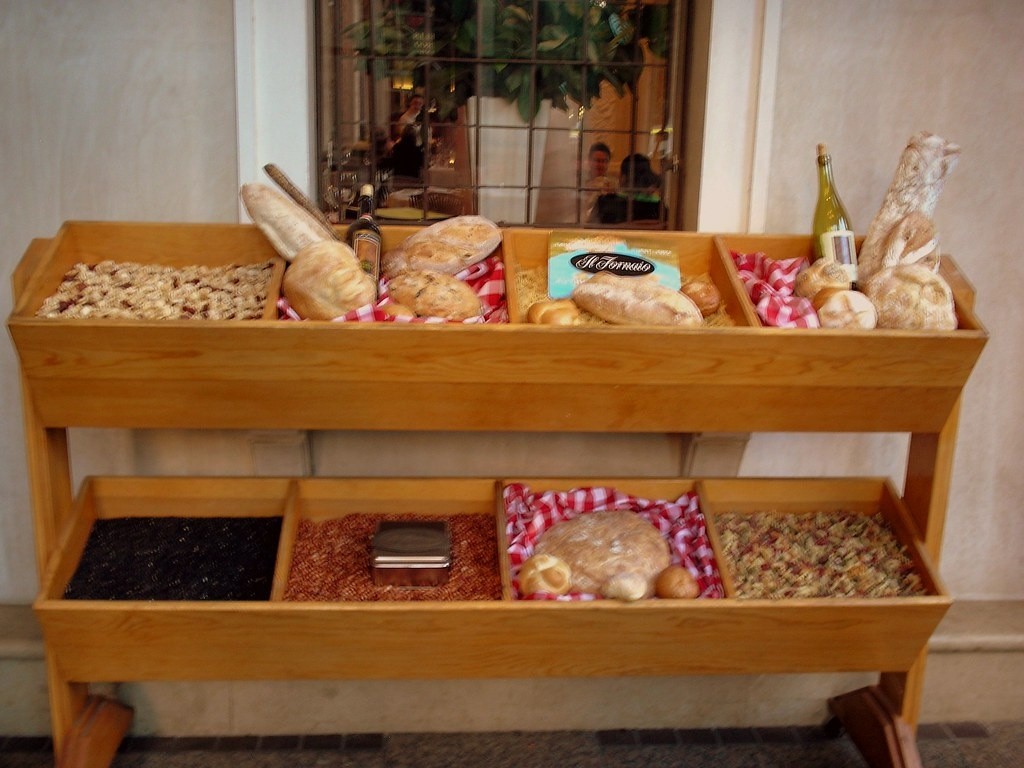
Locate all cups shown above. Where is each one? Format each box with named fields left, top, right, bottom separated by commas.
left=323, top=140, right=386, bottom=219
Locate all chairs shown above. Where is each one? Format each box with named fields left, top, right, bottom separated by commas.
left=410, top=194, right=464, bottom=215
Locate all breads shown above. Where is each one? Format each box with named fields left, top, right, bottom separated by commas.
left=797, top=129, right=962, bottom=329
left=516, top=509, right=700, bottom=599
left=527, top=278, right=722, bottom=329
left=238, top=181, right=499, bottom=322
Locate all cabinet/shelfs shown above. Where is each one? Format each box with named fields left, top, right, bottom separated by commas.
left=5, top=221, right=990, bottom=768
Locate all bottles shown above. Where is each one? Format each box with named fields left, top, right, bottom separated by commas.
left=811, top=142, right=858, bottom=287
left=346, top=183, right=383, bottom=290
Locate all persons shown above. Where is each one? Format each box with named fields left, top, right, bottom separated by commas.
left=583, top=152, right=670, bottom=231
left=395, top=94, right=425, bottom=146
left=646, top=130, right=669, bottom=160
left=580, top=140, right=621, bottom=212
left=352, top=121, right=425, bottom=180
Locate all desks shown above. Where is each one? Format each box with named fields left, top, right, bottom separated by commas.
left=387, top=186, right=456, bottom=209
left=428, top=165, right=454, bottom=187
